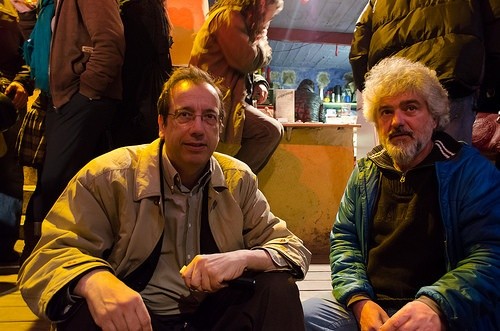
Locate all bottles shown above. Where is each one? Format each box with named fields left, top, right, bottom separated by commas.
left=327, top=90, right=351, bottom=102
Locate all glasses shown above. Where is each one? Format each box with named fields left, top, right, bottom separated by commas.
left=167, top=109, right=223, bottom=126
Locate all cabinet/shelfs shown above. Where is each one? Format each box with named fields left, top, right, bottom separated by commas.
left=322, top=93, right=357, bottom=119
left=258, top=120, right=365, bottom=265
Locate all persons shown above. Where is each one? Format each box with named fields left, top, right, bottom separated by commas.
left=0, top=0, right=172, bottom=269
left=18, top=64, right=312, bottom=331
left=182, top=0, right=285, bottom=177
left=302, top=56, right=500, bottom=331
left=348, top=0, right=500, bottom=146
left=294, top=79, right=326, bottom=123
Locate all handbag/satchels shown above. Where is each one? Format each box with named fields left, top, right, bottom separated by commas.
left=471, top=110, right=500, bottom=153
